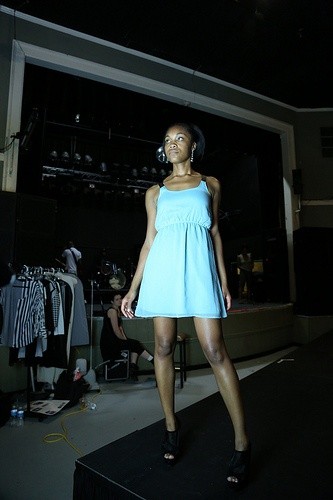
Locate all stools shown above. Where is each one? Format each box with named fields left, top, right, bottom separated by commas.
left=104, top=350, right=130, bottom=383
left=156, top=332, right=188, bottom=389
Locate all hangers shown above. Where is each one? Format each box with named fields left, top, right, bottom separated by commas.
left=12, top=265, right=65, bottom=288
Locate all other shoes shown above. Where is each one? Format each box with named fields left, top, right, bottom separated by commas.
left=130, top=368, right=137, bottom=381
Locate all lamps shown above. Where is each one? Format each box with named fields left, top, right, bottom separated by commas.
left=42, top=151, right=173, bottom=199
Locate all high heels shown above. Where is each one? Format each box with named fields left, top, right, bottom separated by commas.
left=224, top=441, right=252, bottom=488
left=161, top=429, right=181, bottom=466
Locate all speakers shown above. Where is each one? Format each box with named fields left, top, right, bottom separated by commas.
left=292, top=168, right=303, bottom=193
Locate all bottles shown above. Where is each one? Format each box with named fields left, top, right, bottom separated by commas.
left=17, top=407, right=24, bottom=426
left=11, top=405, right=18, bottom=425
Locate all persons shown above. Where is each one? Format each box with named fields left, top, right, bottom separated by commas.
left=231, top=248, right=255, bottom=306
left=120, top=123, right=256, bottom=488
left=100, top=292, right=154, bottom=382
left=61, top=240, right=82, bottom=277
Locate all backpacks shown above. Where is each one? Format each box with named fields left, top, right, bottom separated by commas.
left=53, top=369, right=89, bottom=409
